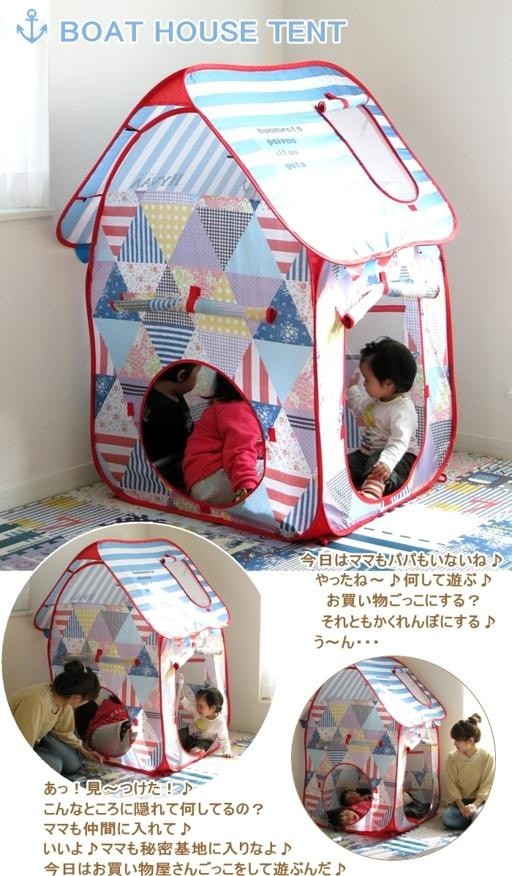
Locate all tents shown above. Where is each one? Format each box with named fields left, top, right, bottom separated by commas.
left=303, top=666, right=439, bottom=829
left=33, top=543, right=232, bottom=768
left=57, top=64, right=455, bottom=540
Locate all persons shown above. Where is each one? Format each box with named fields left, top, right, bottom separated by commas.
left=327, top=802, right=370, bottom=826
left=176, top=687, right=232, bottom=761
left=9, top=661, right=103, bottom=776
left=340, top=788, right=370, bottom=806
left=140, top=359, right=201, bottom=489
left=89, top=695, right=131, bottom=758
left=344, top=337, right=420, bottom=498
left=184, top=372, right=262, bottom=508
left=75, top=690, right=101, bottom=738
left=441, top=713, right=495, bottom=830
